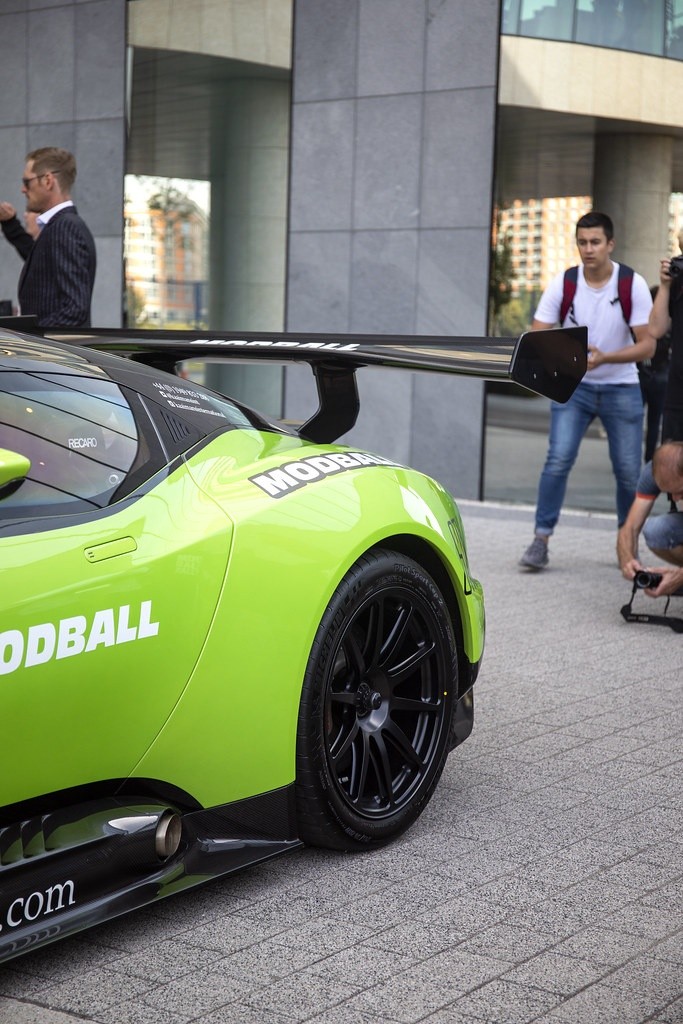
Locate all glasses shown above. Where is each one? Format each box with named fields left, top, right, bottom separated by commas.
left=22, top=170, right=60, bottom=189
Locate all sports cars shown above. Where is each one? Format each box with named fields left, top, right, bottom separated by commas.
left=0, top=324, right=591, bottom=962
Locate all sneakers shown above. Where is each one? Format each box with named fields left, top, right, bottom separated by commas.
left=521, top=537, right=549, bottom=566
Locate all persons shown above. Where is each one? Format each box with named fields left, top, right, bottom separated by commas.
left=0, top=146, right=96, bottom=332
left=518, top=211, right=683, bottom=598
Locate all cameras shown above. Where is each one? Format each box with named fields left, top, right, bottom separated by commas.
left=670, top=257, right=683, bottom=278
left=633, top=570, right=662, bottom=589
